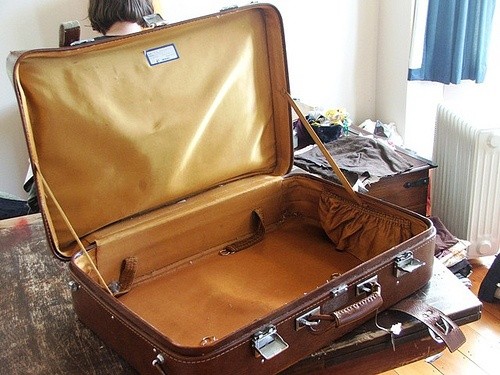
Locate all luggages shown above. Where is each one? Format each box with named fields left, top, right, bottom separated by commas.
left=11, top=1, right=500, bottom=365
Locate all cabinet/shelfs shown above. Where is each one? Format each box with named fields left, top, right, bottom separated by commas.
left=293, top=122, right=438, bottom=215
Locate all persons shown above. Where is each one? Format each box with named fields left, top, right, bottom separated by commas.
left=23, top=0, right=154, bottom=214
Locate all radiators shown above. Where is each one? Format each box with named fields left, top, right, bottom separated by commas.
left=430, top=103, right=500, bottom=258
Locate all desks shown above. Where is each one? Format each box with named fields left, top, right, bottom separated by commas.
left=1, top=165, right=483, bottom=375
